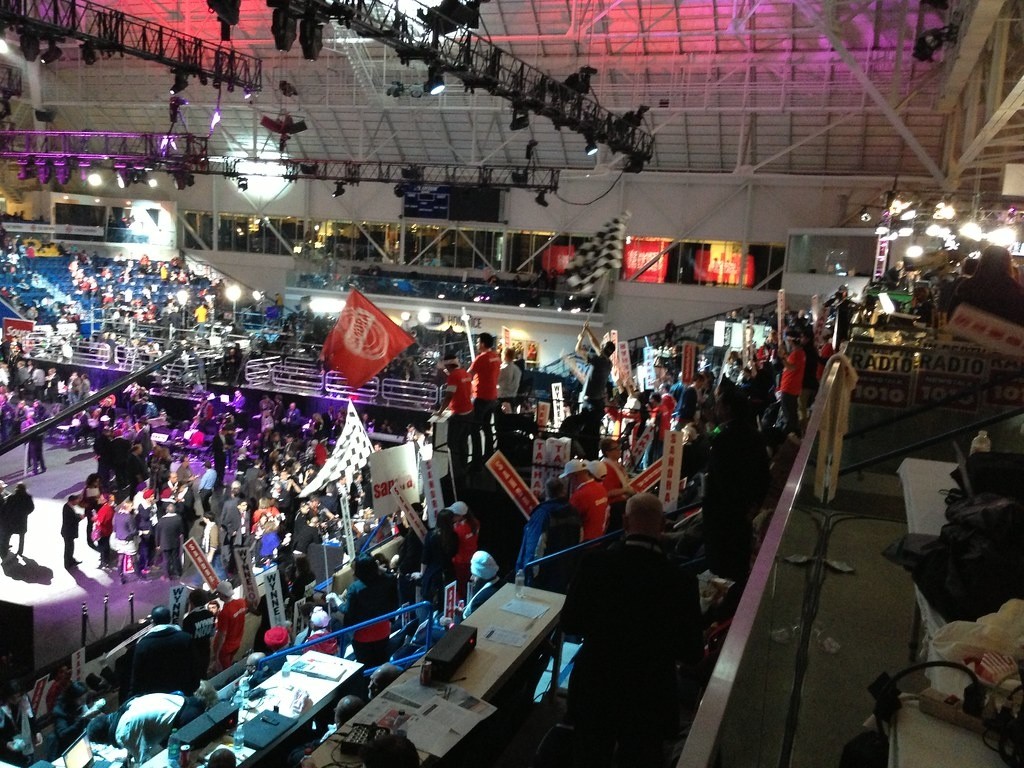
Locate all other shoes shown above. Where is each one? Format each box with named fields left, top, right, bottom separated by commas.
left=67, top=558, right=181, bottom=585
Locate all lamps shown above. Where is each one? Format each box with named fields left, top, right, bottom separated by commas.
left=0, top=1, right=646, bottom=208
left=913, top=22, right=956, bottom=65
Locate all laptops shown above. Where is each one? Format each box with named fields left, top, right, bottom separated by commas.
left=61, top=729, right=111, bottom=768
left=230, top=709, right=299, bottom=750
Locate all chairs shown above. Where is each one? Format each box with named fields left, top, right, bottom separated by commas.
left=0, top=255, right=208, bottom=326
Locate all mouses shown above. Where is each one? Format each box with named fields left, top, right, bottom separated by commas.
left=248, top=687, right=266, bottom=700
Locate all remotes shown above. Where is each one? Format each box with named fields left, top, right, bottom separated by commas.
left=261, top=715, right=280, bottom=726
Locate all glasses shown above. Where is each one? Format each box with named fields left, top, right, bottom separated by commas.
left=609, top=446, right=619, bottom=451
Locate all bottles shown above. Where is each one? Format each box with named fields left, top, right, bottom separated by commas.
left=458, top=599, right=464, bottom=611
left=234, top=691, right=243, bottom=719
left=300, top=749, right=315, bottom=768
left=234, top=728, right=243, bottom=755
left=515, top=570, right=525, bottom=598
left=241, top=680, right=249, bottom=704
left=168, top=728, right=179, bottom=768
left=969, top=431, right=991, bottom=457
left=325, top=532, right=329, bottom=543
left=282, top=662, right=289, bottom=685
left=393, top=710, right=408, bottom=737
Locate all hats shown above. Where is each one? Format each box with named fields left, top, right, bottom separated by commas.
left=265, top=627, right=291, bottom=649
left=217, top=581, right=233, bottom=597
left=583, top=460, right=607, bottom=480
left=310, top=605, right=329, bottom=628
left=0, top=480, right=8, bottom=489
left=143, top=489, right=154, bottom=498
left=359, top=734, right=419, bottom=768
left=470, top=551, right=499, bottom=579
left=161, top=488, right=172, bottom=498
left=247, top=652, right=265, bottom=665
left=151, top=606, right=172, bottom=625
left=445, top=501, right=468, bottom=515
left=558, top=459, right=588, bottom=479
left=100, top=415, right=110, bottom=422
left=136, top=482, right=148, bottom=490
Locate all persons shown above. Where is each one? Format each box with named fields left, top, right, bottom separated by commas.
left=1, top=210, right=1023, bottom=768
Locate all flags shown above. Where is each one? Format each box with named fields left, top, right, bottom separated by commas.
left=321, top=291, right=415, bottom=390
left=564, top=216, right=626, bottom=289
left=299, top=402, right=375, bottom=497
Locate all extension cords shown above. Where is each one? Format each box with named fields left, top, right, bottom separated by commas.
left=918, top=687, right=1024, bottom=746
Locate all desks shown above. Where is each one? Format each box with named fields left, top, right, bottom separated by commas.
left=305, top=542, right=344, bottom=584
left=137, top=649, right=365, bottom=768
left=897, top=457, right=975, bottom=700
left=300, top=583, right=568, bottom=768
